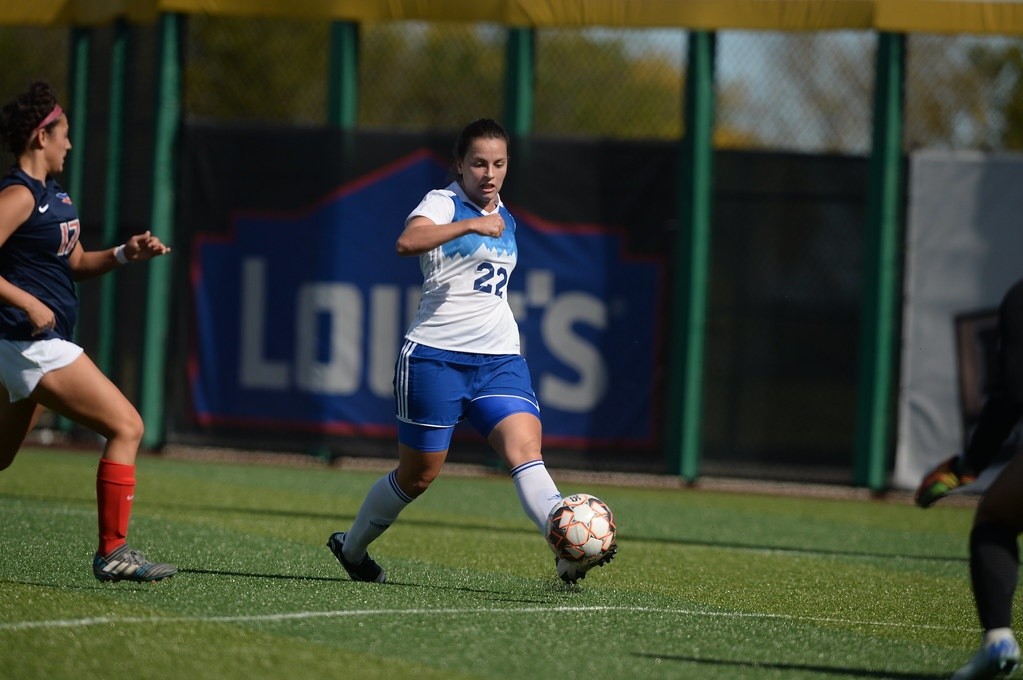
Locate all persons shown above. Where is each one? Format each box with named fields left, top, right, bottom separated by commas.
left=326, top=116, right=620, bottom=585
left=914, top=277, right=1023, bottom=680
left=0, top=82, right=184, bottom=582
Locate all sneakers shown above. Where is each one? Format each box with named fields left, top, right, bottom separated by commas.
left=328, top=532, right=384, bottom=583
left=555, top=543, right=619, bottom=583
left=93, top=544, right=178, bottom=582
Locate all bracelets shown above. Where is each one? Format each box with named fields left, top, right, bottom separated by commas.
left=113, top=244, right=129, bottom=264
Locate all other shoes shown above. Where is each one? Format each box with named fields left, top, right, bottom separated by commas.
left=951, top=626, right=1022, bottom=680
left=913, top=456, right=977, bottom=508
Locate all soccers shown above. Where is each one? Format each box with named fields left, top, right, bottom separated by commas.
left=542, top=492, right=618, bottom=568
